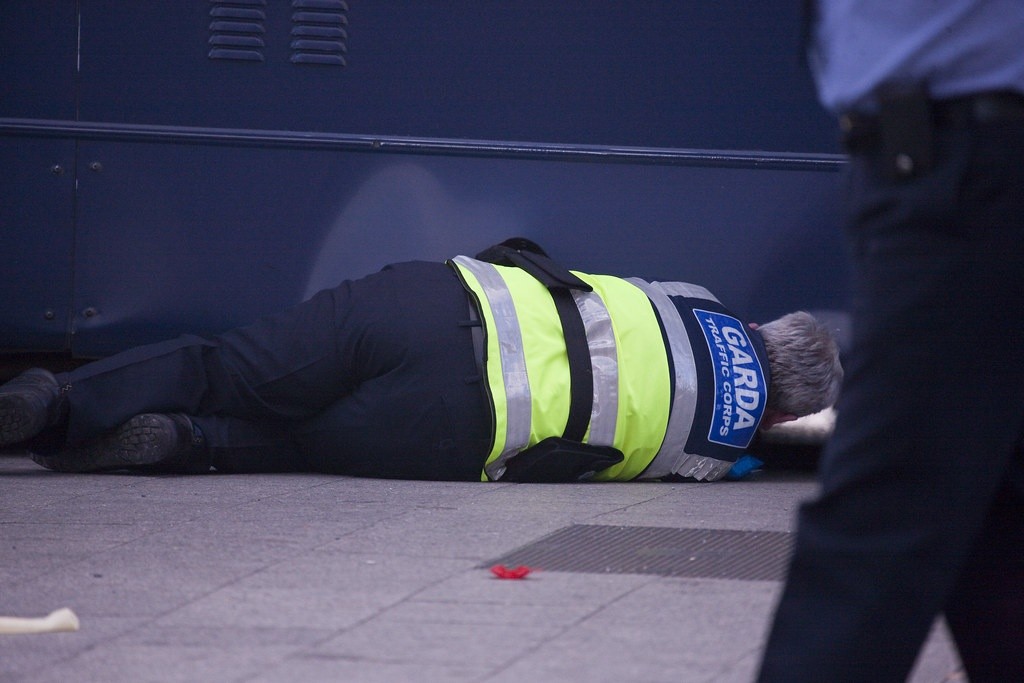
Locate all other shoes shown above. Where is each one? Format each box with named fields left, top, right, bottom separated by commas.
left=0, top=368, right=65, bottom=442
left=29, top=412, right=199, bottom=475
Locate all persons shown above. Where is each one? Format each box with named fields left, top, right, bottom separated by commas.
left=0, top=234, right=850, bottom=482
left=752, top=0, right=1024, bottom=683
